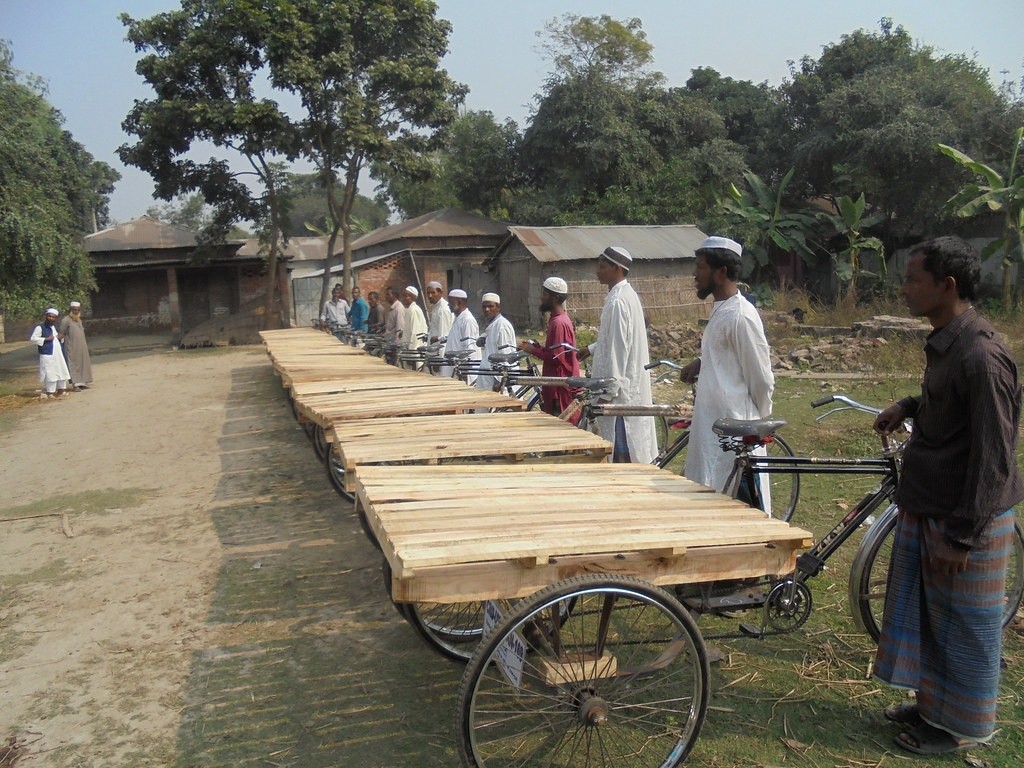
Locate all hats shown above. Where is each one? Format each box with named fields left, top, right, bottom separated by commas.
left=601, top=246, right=632, bottom=270
left=543, top=277, right=567, bottom=293
left=405, top=286, right=418, bottom=297
left=428, top=282, right=442, bottom=290
left=449, top=289, right=467, bottom=298
left=70, top=302, right=80, bottom=307
left=481, top=293, right=500, bottom=304
left=695, top=236, right=743, bottom=258
left=44, top=309, right=59, bottom=316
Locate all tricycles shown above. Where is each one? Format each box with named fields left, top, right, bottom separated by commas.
left=390, top=392, right=1023, bottom=768
left=271, top=316, right=808, bottom=539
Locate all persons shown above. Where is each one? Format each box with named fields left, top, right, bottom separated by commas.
left=30, top=308, right=71, bottom=399
left=320, top=283, right=523, bottom=415
left=872, top=236, right=1024, bottom=752
left=59, top=301, right=93, bottom=392
left=575, top=247, right=661, bottom=468
left=520, top=277, right=584, bottom=425
left=684, top=234, right=775, bottom=618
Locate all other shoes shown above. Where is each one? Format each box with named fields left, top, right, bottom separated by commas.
left=718, top=609, right=753, bottom=618
left=48, top=394, right=55, bottom=399
left=72, top=385, right=91, bottom=392
left=885, top=704, right=977, bottom=754
left=58, top=391, right=70, bottom=396
